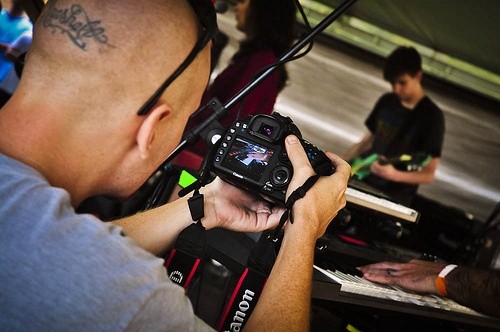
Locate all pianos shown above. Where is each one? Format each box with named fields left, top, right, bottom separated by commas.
left=172, top=227, right=500, bottom=330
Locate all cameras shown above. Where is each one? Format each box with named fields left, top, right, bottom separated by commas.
left=212, top=112, right=335, bottom=208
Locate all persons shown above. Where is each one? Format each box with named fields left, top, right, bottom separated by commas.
left=180, top=1, right=299, bottom=157
left=329, top=46, right=446, bottom=206
left=1, top=0, right=351, bottom=332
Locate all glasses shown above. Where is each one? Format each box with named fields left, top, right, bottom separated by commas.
left=137, top=0, right=217, bottom=116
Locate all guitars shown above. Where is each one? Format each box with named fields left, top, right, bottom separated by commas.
left=349, top=153, right=413, bottom=180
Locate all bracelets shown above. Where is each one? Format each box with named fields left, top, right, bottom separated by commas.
left=437, top=262, right=458, bottom=297
